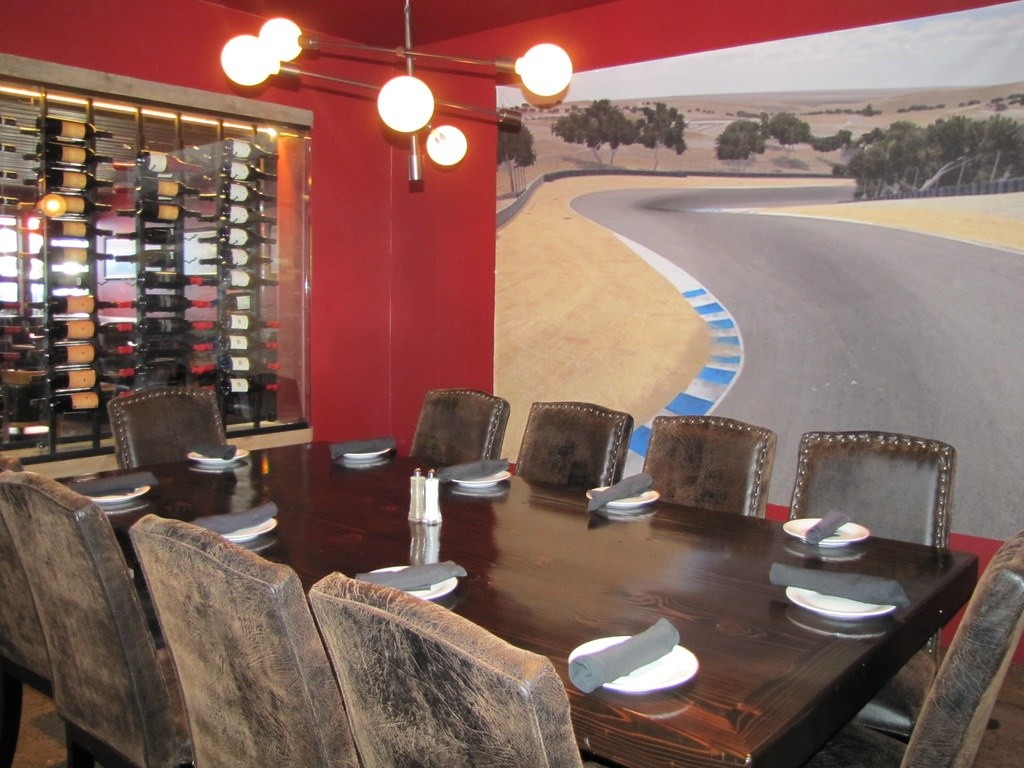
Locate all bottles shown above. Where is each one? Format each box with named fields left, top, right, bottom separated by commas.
left=25, top=273, right=115, bottom=414
left=113, top=150, right=202, bottom=266
left=198, top=206, right=279, bottom=289
left=18, top=116, right=114, bottom=261
left=0, top=117, right=22, bottom=359
left=408, top=468, right=426, bottom=523
left=200, top=138, right=279, bottom=204
left=197, top=356, right=280, bottom=398
left=116, top=270, right=201, bottom=397
left=422, top=468, right=443, bottom=524
left=197, top=293, right=279, bottom=354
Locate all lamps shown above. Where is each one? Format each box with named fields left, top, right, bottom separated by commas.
left=222, top=0, right=573, bottom=181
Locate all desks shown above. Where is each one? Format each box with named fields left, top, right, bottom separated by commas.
left=55, top=441, right=978, bottom=768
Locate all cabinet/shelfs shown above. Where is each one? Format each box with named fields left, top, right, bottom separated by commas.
left=0, top=52, right=315, bottom=466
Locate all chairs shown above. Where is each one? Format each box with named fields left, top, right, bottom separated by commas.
left=801, top=531, right=1024, bottom=768
left=406, top=388, right=510, bottom=463
left=107, top=386, right=227, bottom=469
left=642, top=415, right=777, bottom=518
left=0, top=454, right=582, bottom=768
left=789, top=431, right=957, bottom=739
left=515, top=401, right=635, bottom=490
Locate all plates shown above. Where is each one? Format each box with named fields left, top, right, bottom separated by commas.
left=372, top=566, right=458, bottom=599
left=783, top=518, right=870, bottom=546
left=221, top=518, right=278, bottom=541
left=188, top=449, right=249, bottom=465
left=786, top=585, right=896, bottom=618
left=586, top=486, right=660, bottom=508
left=568, top=635, right=699, bottom=692
left=89, top=485, right=151, bottom=503
left=452, top=470, right=511, bottom=487
left=343, top=448, right=391, bottom=460
